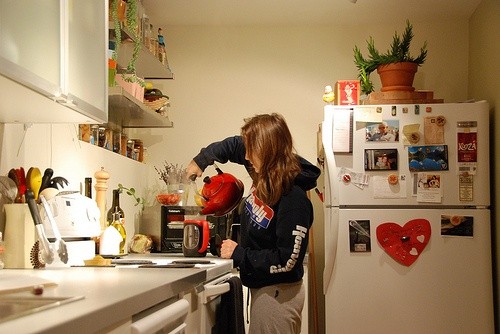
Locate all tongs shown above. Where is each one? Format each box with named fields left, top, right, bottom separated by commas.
left=26, top=187, right=69, bottom=265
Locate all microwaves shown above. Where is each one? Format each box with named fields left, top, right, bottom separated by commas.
left=160, top=206, right=234, bottom=254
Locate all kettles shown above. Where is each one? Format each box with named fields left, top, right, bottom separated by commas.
left=190, top=163, right=244, bottom=218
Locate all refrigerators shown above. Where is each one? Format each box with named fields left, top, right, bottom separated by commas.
left=313, top=104, right=497, bottom=334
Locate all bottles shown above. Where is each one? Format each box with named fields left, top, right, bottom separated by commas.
left=84, top=177, right=92, bottom=199
left=80, top=122, right=143, bottom=163
left=107, top=190, right=125, bottom=226
left=108, top=206, right=126, bottom=252
left=149, top=24, right=159, bottom=59
left=177, top=184, right=185, bottom=206
left=158, top=27, right=167, bottom=66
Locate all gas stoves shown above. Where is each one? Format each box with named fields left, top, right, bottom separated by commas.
left=41, top=194, right=234, bottom=284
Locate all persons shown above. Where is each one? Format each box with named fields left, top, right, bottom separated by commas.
left=375, top=156, right=392, bottom=169
left=186, top=113, right=321, bottom=334
left=367, top=122, right=395, bottom=141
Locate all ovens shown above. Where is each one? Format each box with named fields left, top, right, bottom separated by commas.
left=202, top=272, right=240, bottom=334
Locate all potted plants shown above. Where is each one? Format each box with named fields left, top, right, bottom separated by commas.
left=353, top=18, right=429, bottom=96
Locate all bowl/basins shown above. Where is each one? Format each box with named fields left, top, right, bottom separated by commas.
left=156, top=193, right=182, bottom=206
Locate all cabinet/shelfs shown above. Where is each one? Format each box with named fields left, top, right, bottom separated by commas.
left=0, top=0, right=110, bottom=125
left=108, top=20, right=174, bottom=128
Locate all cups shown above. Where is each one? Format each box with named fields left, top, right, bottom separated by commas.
left=183, top=214, right=209, bottom=257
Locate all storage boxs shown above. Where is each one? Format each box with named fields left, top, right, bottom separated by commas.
left=114, top=73, right=144, bottom=103
left=334, top=80, right=360, bottom=106
